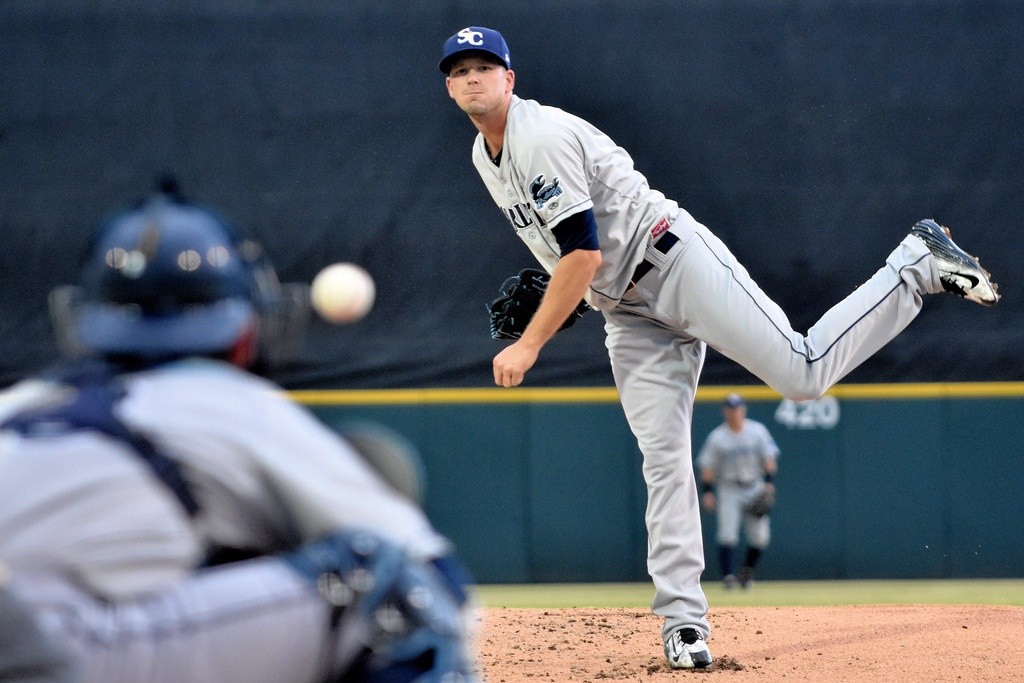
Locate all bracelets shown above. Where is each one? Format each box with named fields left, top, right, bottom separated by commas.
left=764, top=472, right=776, bottom=486
left=698, top=482, right=715, bottom=495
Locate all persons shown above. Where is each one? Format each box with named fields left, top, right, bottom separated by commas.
left=1, top=199, right=474, bottom=683
left=694, top=392, right=782, bottom=592
left=439, top=25, right=1003, bottom=670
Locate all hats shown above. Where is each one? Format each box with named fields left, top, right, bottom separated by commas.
left=439, top=26, right=510, bottom=76
left=73, top=200, right=250, bottom=355
left=723, top=395, right=744, bottom=408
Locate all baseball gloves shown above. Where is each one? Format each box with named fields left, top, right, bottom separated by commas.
left=483, top=268, right=593, bottom=342
left=747, top=492, right=776, bottom=519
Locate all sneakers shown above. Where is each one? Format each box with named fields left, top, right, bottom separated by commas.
left=910, top=219, right=1001, bottom=306
left=664, top=628, right=712, bottom=670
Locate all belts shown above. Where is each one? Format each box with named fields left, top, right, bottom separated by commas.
left=623, top=231, right=680, bottom=295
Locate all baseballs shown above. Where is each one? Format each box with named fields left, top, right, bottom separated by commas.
left=308, top=261, right=377, bottom=328
